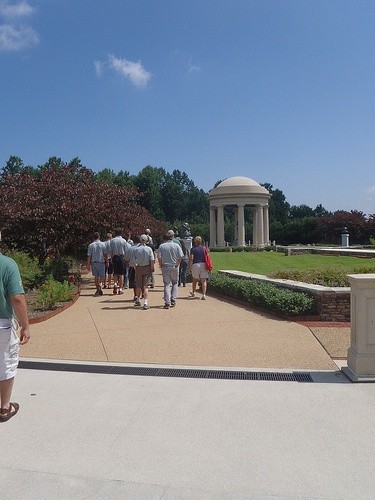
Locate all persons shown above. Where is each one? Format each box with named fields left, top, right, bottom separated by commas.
left=180, top=223, right=191, bottom=239
left=132, top=234, right=154, bottom=309
left=188, top=236, right=208, bottom=300
left=157, top=230, right=184, bottom=309
left=87, top=232, right=109, bottom=295
left=174, top=227, right=179, bottom=237
left=103, top=229, right=190, bottom=289
left=110, top=227, right=129, bottom=294
left=0, top=254, right=31, bottom=422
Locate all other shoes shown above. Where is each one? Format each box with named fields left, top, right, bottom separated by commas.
left=171, top=302, right=176, bottom=305
left=98, top=285, right=103, bottom=295
left=164, top=305, right=170, bottom=309
left=143, top=304, right=148, bottom=310
left=202, top=296, right=206, bottom=301
left=95, top=291, right=99, bottom=294
left=113, top=285, right=117, bottom=295
left=118, top=290, right=124, bottom=295
left=134, top=301, right=141, bottom=306
left=190, top=291, right=195, bottom=297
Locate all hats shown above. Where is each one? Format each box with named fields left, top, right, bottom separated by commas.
left=139, top=234, right=148, bottom=242
left=166, top=230, right=175, bottom=237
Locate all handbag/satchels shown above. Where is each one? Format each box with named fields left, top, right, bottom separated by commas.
left=203, top=247, right=213, bottom=272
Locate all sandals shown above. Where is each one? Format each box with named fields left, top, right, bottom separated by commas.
left=0, top=403, right=19, bottom=421
